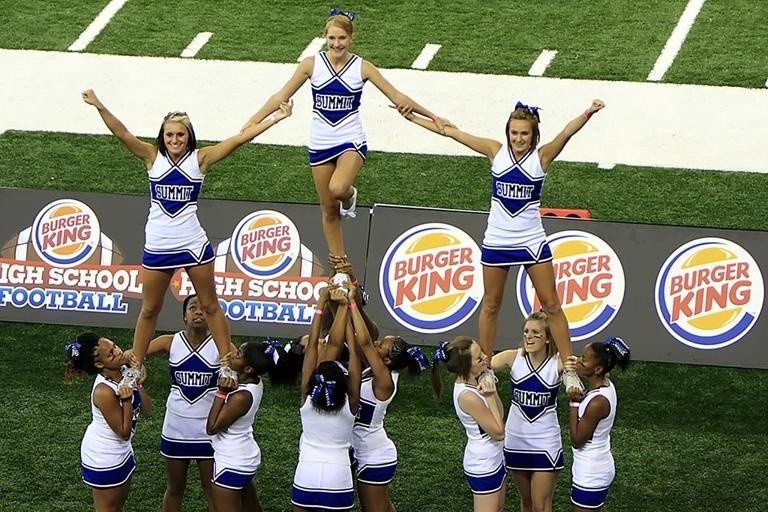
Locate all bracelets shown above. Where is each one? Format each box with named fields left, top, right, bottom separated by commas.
left=584, top=110, right=593, bottom=119
left=271, top=115, right=279, bottom=125
left=569, top=398, right=580, bottom=407
left=136, top=383, right=143, bottom=389
left=431, top=113, right=438, bottom=121
left=214, top=389, right=227, bottom=401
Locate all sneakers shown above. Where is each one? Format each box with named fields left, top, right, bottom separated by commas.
left=562, top=370, right=584, bottom=394
left=477, top=367, right=497, bottom=393
left=116, top=363, right=148, bottom=396
left=217, top=363, right=238, bottom=388
left=339, top=185, right=357, bottom=223
left=330, top=272, right=350, bottom=301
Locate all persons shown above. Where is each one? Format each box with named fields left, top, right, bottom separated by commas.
left=565, top=336, right=631, bottom=512
left=240, top=6, right=458, bottom=297
left=206, top=336, right=282, bottom=512
left=83, top=89, right=294, bottom=396
left=389, top=98, right=606, bottom=394
left=64, top=333, right=152, bottom=512
left=431, top=335, right=508, bottom=512
left=482, top=307, right=579, bottom=512
left=283, top=281, right=430, bottom=512
left=123, top=294, right=238, bottom=512
